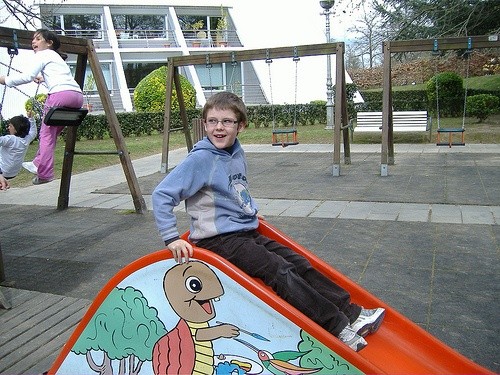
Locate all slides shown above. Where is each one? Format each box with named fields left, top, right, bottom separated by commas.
left=195, top=218, right=500, bottom=375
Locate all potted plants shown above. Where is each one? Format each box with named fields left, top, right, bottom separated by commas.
left=214, top=4, right=230, bottom=47
left=184, top=19, right=205, bottom=47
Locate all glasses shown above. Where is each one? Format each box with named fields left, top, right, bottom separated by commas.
left=206, top=119, right=241, bottom=125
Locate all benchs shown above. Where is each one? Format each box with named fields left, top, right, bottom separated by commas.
left=349, top=110, right=433, bottom=143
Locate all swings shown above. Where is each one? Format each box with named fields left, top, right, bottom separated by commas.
left=0, top=62, right=88, bottom=127
left=265, top=56, right=301, bottom=148
left=0, top=47, right=43, bottom=137
left=430, top=49, right=472, bottom=148
left=206, top=60, right=238, bottom=97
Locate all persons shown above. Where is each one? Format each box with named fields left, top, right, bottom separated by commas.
left=0, top=29, right=83, bottom=184
left=0, top=110, right=37, bottom=189
left=152, top=93, right=385, bottom=353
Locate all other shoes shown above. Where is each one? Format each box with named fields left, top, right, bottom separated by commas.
left=21, top=162, right=39, bottom=174
left=32, top=175, right=55, bottom=184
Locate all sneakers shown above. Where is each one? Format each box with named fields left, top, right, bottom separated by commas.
left=350, top=306, right=386, bottom=339
left=338, top=323, right=368, bottom=352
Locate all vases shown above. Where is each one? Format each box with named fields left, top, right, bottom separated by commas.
left=163, top=44, right=171, bottom=48
